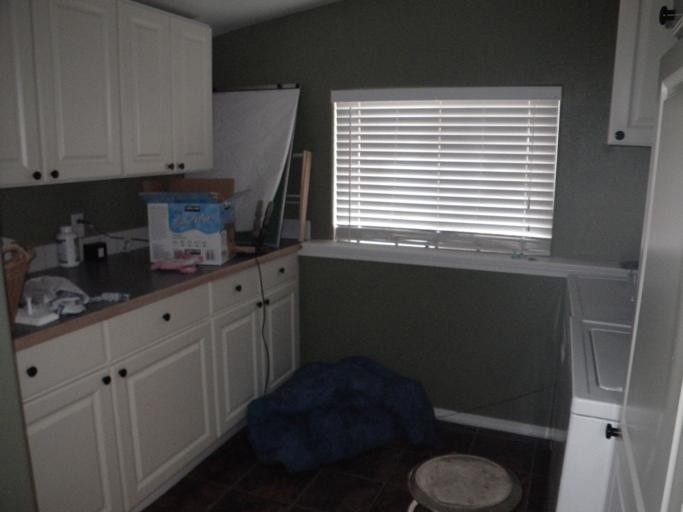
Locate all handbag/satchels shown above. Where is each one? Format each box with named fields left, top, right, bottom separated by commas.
left=246, top=358, right=435, bottom=475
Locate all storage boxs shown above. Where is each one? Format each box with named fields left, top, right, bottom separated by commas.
left=138, top=176, right=245, bottom=267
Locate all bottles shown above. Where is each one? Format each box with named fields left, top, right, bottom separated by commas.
left=55, top=224, right=80, bottom=269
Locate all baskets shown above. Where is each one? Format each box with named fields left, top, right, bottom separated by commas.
left=2, top=233, right=36, bottom=331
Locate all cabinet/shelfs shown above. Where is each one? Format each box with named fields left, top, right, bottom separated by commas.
left=122, top=0, right=214, bottom=178
left=9, top=282, right=218, bottom=511
left=545, top=277, right=635, bottom=511
left=208, top=251, right=302, bottom=440
left=0, top=0, right=122, bottom=189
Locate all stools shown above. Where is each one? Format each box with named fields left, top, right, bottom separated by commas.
left=405, top=453, right=525, bottom=511
